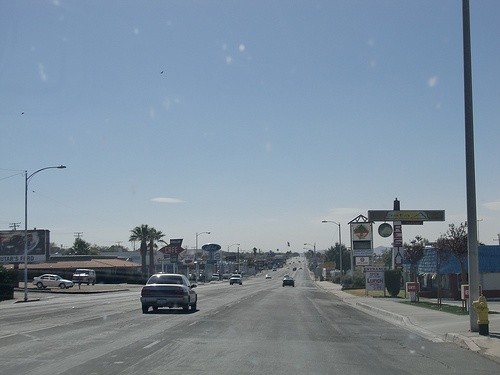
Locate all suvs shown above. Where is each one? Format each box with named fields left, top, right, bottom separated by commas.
left=72, top=268, right=96, bottom=286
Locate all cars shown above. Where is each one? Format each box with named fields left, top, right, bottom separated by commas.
left=228, top=275, right=242, bottom=285
left=282, top=276, right=295, bottom=287
left=31, top=273, right=73, bottom=289
left=139, top=273, right=197, bottom=314
left=264, top=255, right=303, bottom=279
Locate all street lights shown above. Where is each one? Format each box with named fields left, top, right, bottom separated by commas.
left=23, top=164, right=67, bottom=300
left=322, top=220, right=343, bottom=285
left=227, top=243, right=241, bottom=280
left=195, top=231, right=210, bottom=284
left=303, top=242, right=317, bottom=282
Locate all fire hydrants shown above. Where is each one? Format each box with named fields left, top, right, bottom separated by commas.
left=472, top=295, right=490, bottom=335
